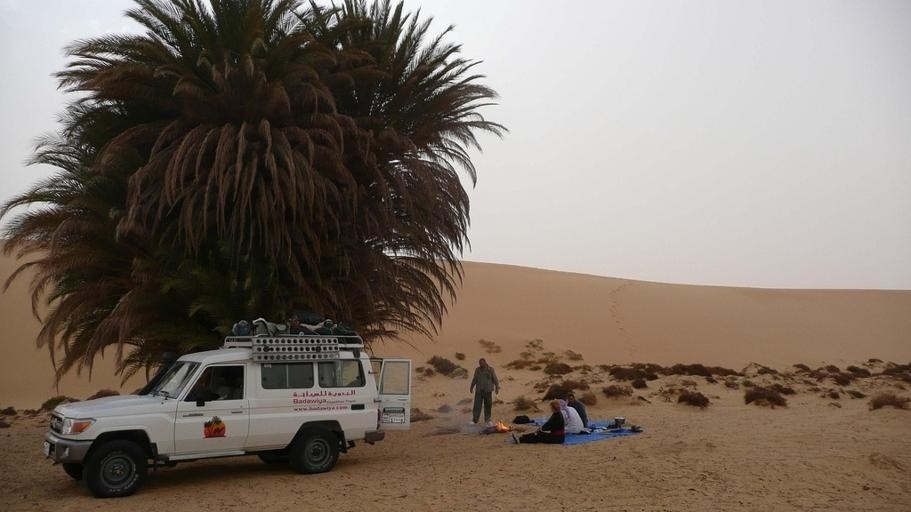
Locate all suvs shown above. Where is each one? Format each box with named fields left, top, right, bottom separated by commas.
left=43, top=317, right=415, bottom=500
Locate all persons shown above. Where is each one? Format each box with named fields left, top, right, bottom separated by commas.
left=566, top=394, right=589, bottom=426
left=561, top=401, right=584, bottom=435
left=511, top=400, right=565, bottom=444
left=470, top=358, right=501, bottom=425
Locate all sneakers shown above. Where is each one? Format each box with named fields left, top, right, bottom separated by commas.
left=512, top=433, right=520, bottom=444
